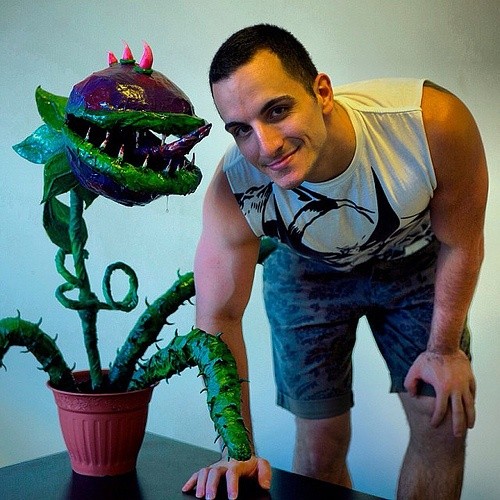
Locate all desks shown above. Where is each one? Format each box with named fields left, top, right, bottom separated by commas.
left=0, top=432, right=392, bottom=500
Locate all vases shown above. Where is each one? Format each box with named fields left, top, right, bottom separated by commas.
left=46, top=369, right=153, bottom=476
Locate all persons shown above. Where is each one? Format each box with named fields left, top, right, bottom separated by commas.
left=181, top=24, right=488, bottom=500
